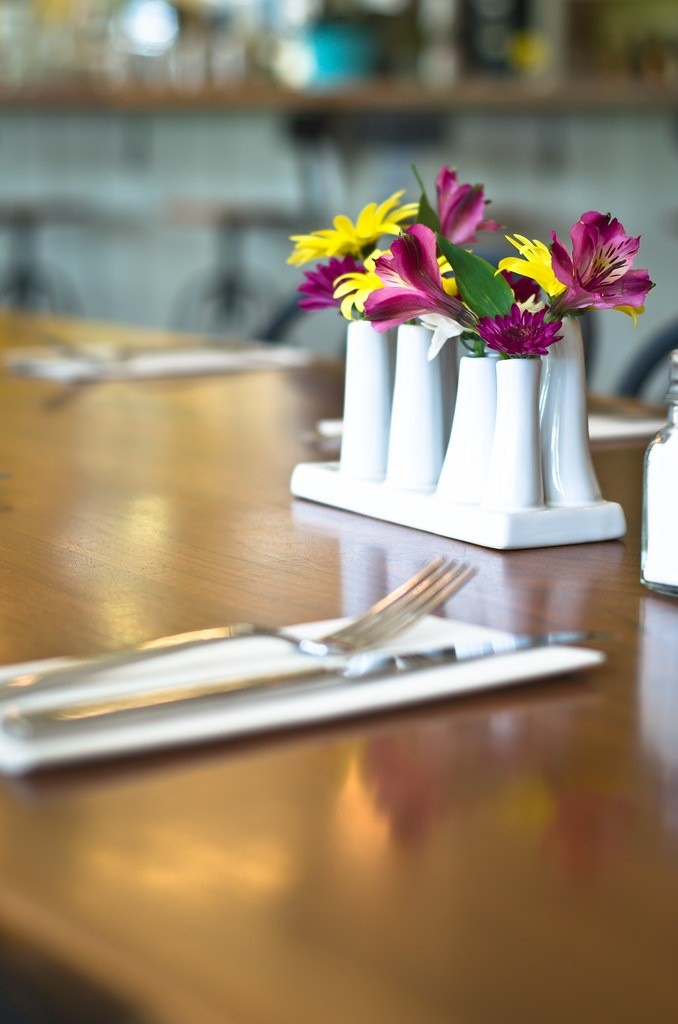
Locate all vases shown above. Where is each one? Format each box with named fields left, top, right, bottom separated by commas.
left=290, top=316, right=630, bottom=551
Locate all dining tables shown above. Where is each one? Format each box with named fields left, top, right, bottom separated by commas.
left=1, top=300, right=678, bottom=1024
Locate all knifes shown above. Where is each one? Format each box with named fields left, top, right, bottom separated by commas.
left=0, top=627, right=609, bottom=740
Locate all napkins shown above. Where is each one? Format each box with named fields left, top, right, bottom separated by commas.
left=318, top=416, right=674, bottom=438
left=3, top=615, right=606, bottom=778
left=12, top=346, right=312, bottom=386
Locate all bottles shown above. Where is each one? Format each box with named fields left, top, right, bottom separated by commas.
left=640, top=351, right=678, bottom=598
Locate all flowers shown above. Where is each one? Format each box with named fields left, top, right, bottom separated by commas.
left=284, top=162, right=656, bottom=362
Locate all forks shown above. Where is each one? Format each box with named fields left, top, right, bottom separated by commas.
left=0, top=556, right=478, bottom=699
